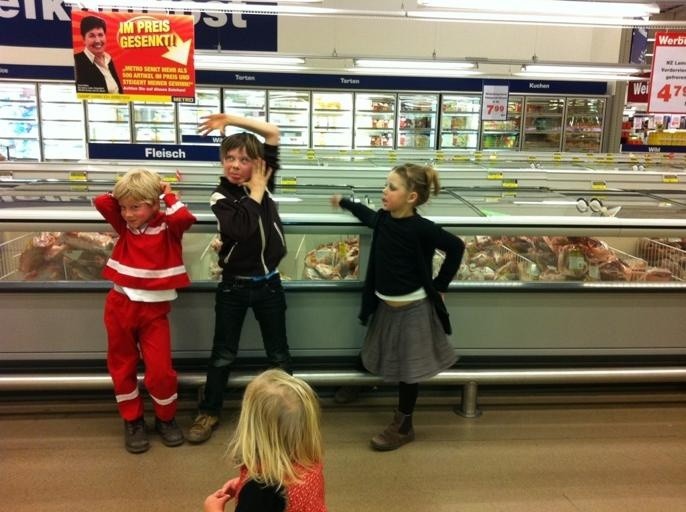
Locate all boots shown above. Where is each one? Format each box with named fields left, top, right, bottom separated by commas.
left=334, top=385, right=361, bottom=403
left=371, top=408, right=416, bottom=451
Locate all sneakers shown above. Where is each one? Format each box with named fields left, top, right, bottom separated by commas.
left=154, top=418, right=183, bottom=446
left=124, top=416, right=150, bottom=453
left=185, top=413, right=219, bottom=443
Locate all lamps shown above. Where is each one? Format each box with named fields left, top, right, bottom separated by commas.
left=353, top=59, right=481, bottom=70
left=520, top=65, right=643, bottom=76
left=193, top=55, right=306, bottom=66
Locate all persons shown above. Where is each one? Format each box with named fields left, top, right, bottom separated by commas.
left=334, top=165, right=466, bottom=452
left=188, top=114, right=293, bottom=444
left=95, top=167, right=196, bottom=452
left=75, top=16, right=121, bottom=93
left=203, top=368, right=325, bottom=512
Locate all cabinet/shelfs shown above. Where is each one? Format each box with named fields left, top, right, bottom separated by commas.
left=0, top=70, right=626, bottom=154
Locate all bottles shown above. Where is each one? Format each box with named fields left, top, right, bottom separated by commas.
left=646, top=131, right=686, bottom=146
